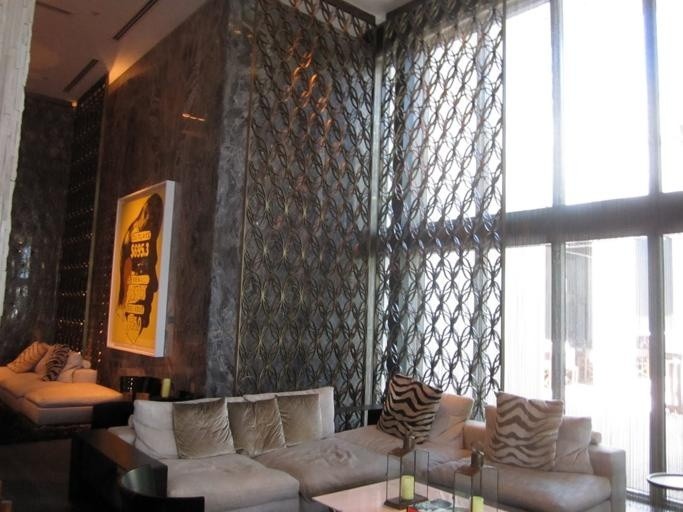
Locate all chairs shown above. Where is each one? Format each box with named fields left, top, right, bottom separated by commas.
left=91, top=376, right=172, bottom=430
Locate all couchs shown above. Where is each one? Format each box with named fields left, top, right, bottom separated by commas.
left=68, top=384, right=625, bottom=511
left=0, top=342, right=123, bottom=434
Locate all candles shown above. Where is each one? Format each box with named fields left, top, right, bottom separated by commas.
left=160, top=378, right=171, bottom=398
left=468, top=496, right=484, bottom=511
left=398, top=473, right=413, bottom=501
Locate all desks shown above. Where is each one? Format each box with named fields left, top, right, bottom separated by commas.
left=645, top=472, right=683, bottom=491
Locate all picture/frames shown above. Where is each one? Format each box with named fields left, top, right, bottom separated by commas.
left=105, top=178, right=175, bottom=361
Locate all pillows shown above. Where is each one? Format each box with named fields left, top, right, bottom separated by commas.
left=493, top=390, right=567, bottom=473
left=373, top=373, right=443, bottom=447
left=273, top=392, right=322, bottom=445
left=5, top=338, right=96, bottom=387
left=225, top=398, right=285, bottom=456
left=167, top=398, right=235, bottom=458
